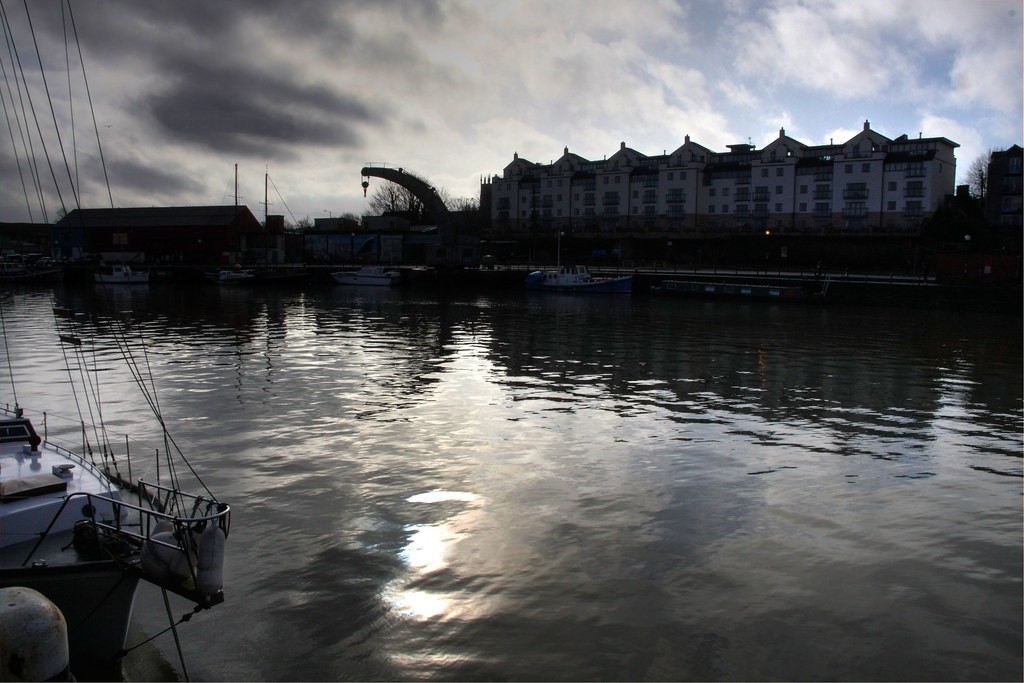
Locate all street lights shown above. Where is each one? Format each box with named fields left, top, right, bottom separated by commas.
left=786, top=149, right=801, bottom=230
left=871, top=144, right=891, bottom=233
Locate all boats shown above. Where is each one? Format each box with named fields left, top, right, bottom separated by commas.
left=2, top=248, right=264, bottom=288
left=524, top=263, right=642, bottom=295
left=329, top=264, right=401, bottom=288
left=1, top=1, right=234, bottom=683
